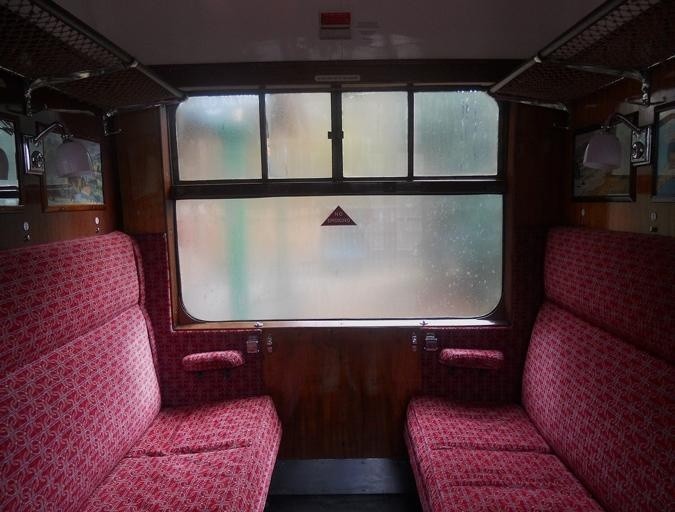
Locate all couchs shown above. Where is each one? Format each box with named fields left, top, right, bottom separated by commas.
left=0, top=229, right=285, bottom=512
left=399, top=221, right=673, bottom=510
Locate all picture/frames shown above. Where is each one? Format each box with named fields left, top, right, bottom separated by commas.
left=570, top=100, right=675, bottom=205
left=0, top=112, right=106, bottom=215
